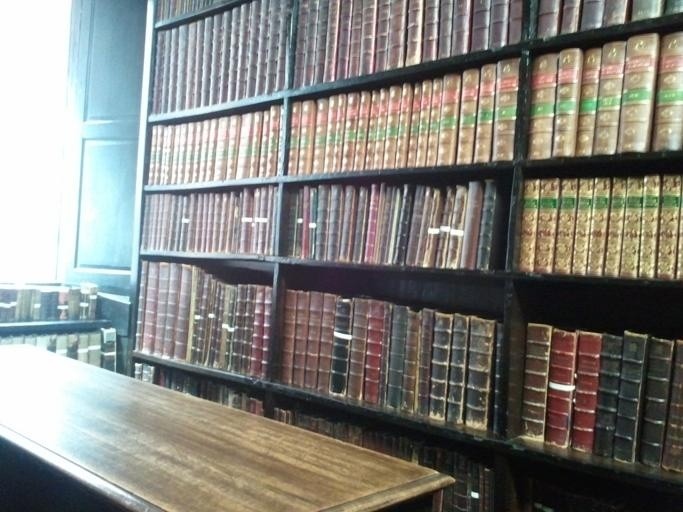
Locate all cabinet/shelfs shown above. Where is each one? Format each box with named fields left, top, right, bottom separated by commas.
left=128, top=0, right=683, bottom=501
left=0, top=282, right=125, bottom=374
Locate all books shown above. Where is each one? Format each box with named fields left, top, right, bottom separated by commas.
left=292, top=0, right=524, bottom=89
left=97, top=291, right=132, bottom=338
left=146, top=103, right=282, bottom=186
left=274, top=405, right=498, bottom=512
left=535, top=0, right=683, bottom=42
left=279, top=287, right=507, bottom=439
left=140, top=186, right=278, bottom=258
left=0, top=282, right=99, bottom=324
left=531, top=479, right=629, bottom=512
left=151, top=1, right=293, bottom=116
left=526, top=30, right=683, bottom=161
left=134, top=360, right=266, bottom=418
left=286, top=58, right=522, bottom=177
left=519, top=321, right=683, bottom=476
left=154, top=0, right=227, bottom=25
left=135, top=260, right=275, bottom=383
left=517, top=173, right=683, bottom=283
left=286, top=177, right=506, bottom=272
left=0, top=327, right=117, bottom=373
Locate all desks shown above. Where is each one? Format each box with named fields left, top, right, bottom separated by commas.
left=0, top=341, right=455, bottom=512
